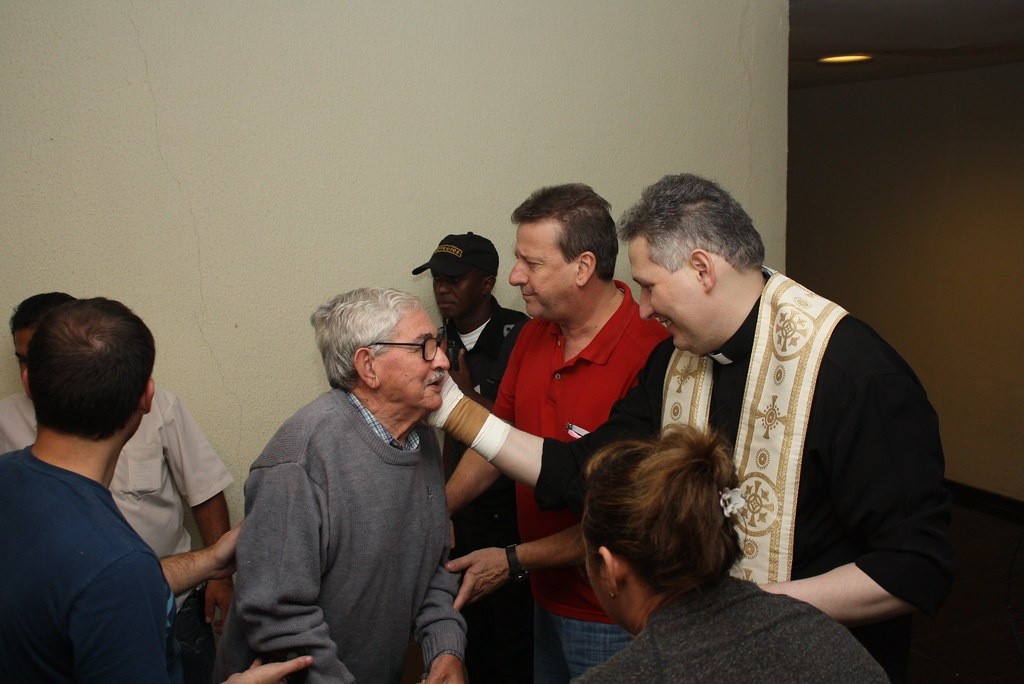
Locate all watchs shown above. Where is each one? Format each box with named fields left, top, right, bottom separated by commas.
left=505, top=544, right=530, bottom=582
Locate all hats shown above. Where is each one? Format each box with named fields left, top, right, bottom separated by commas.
left=412, top=232, right=500, bottom=276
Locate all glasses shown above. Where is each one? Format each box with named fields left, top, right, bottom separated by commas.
left=364, top=332, right=442, bottom=362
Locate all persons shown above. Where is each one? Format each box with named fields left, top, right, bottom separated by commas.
left=445, top=181, right=671, bottom=684
left=427, top=173, right=949, bottom=684
left=213, top=287, right=468, bottom=684
left=568, top=423, right=891, bottom=684
left=0, top=292, right=237, bottom=684
left=0, top=297, right=313, bottom=684
left=412, top=231, right=532, bottom=684
